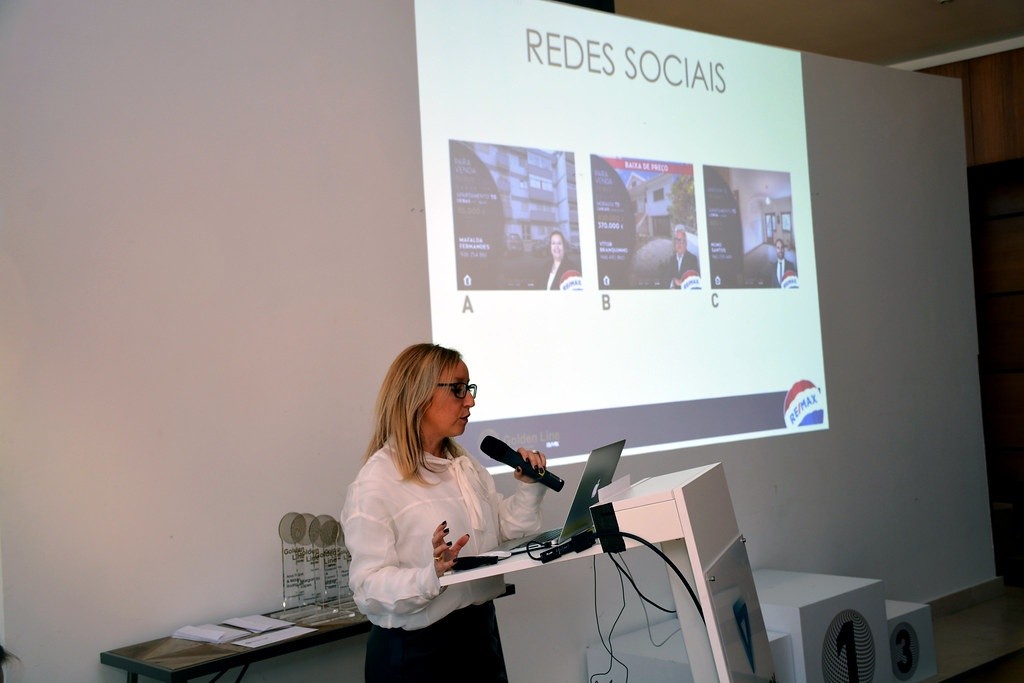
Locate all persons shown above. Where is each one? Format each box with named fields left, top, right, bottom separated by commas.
left=770, top=239, right=794, bottom=288
left=527, top=231, right=579, bottom=291
left=340, top=343, right=548, bottom=683
left=665, top=223, right=697, bottom=289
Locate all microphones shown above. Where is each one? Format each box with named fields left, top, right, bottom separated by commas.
left=480, top=435, right=564, bottom=492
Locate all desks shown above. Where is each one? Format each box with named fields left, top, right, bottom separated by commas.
left=100, top=583, right=516, bottom=683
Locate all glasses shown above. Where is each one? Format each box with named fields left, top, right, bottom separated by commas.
left=434, top=381, right=477, bottom=399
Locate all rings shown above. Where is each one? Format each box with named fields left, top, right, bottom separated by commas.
left=533, top=450, right=539, bottom=454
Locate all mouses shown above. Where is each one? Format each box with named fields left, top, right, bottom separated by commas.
left=479, top=549, right=511, bottom=562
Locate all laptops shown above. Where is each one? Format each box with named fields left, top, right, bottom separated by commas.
left=490, top=440, right=626, bottom=554
